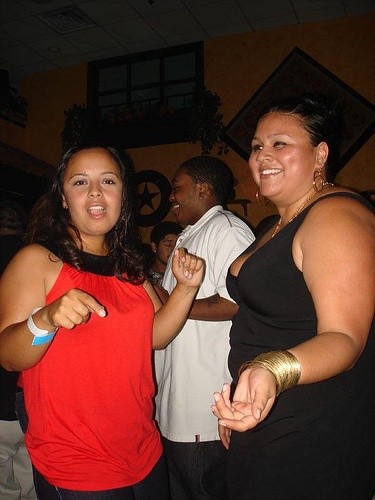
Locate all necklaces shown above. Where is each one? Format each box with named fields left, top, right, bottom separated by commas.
left=273, top=178, right=334, bottom=240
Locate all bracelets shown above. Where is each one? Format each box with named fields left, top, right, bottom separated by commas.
left=239, top=349, right=302, bottom=396
left=28, top=306, right=60, bottom=345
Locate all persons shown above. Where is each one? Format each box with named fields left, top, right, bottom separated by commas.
left=147, top=154, right=258, bottom=500
left=142, top=219, right=186, bottom=286
left=0, top=139, right=206, bottom=500
left=153, top=95, right=375, bottom=500
